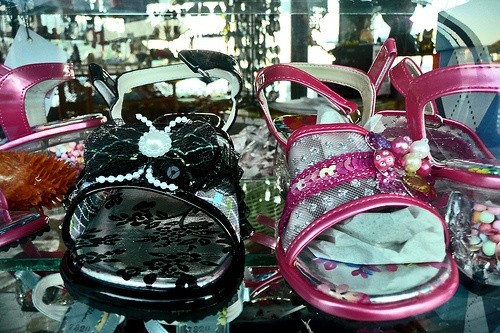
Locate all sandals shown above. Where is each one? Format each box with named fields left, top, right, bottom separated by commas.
left=0, top=191, right=50, bottom=246
left=256, top=38, right=460, bottom=321
left=59, top=50, right=255, bottom=324
left=243, top=216, right=283, bottom=303
left=14, top=270, right=242, bottom=333
left=373, top=57, right=500, bottom=286
left=0, top=62, right=108, bottom=150
